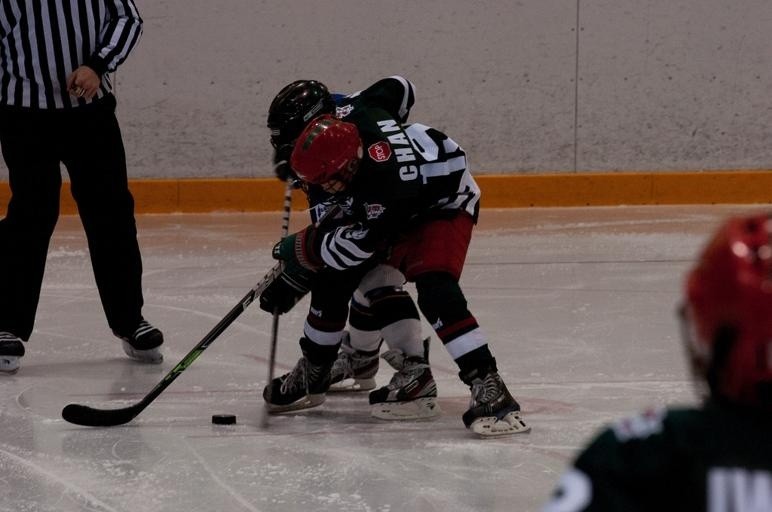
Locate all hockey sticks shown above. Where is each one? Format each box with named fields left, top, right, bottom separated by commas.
left=259, top=178, right=295, bottom=429
left=62, top=199, right=341, bottom=427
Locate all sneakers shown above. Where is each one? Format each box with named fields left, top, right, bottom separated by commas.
left=112, top=321, right=163, bottom=350
left=0, top=332, right=24, bottom=356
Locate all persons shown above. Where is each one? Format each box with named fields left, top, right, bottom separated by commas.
left=263, top=113, right=530, bottom=435
left=1, top=1, right=163, bottom=376
left=262, top=66, right=439, bottom=420
left=544, top=207, right=770, bottom=511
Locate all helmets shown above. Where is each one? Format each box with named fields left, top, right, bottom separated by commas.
left=679, top=209, right=772, bottom=387
left=267, top=80, right=336, bottom=149
left=290, top=114, right=364, bottom=206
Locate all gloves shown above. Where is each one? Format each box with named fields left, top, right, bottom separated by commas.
left=260, top=224, right=327, bottom=316
left=273, top=144, right=300, bottom=189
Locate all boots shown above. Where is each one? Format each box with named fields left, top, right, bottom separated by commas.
left=459, top=357, right=520, bottom=428
left=263, top=337, right=342, bottom=404
left=330, top=331, right=384, bottom=385
left=369, top=337, right=437, bottom=404
